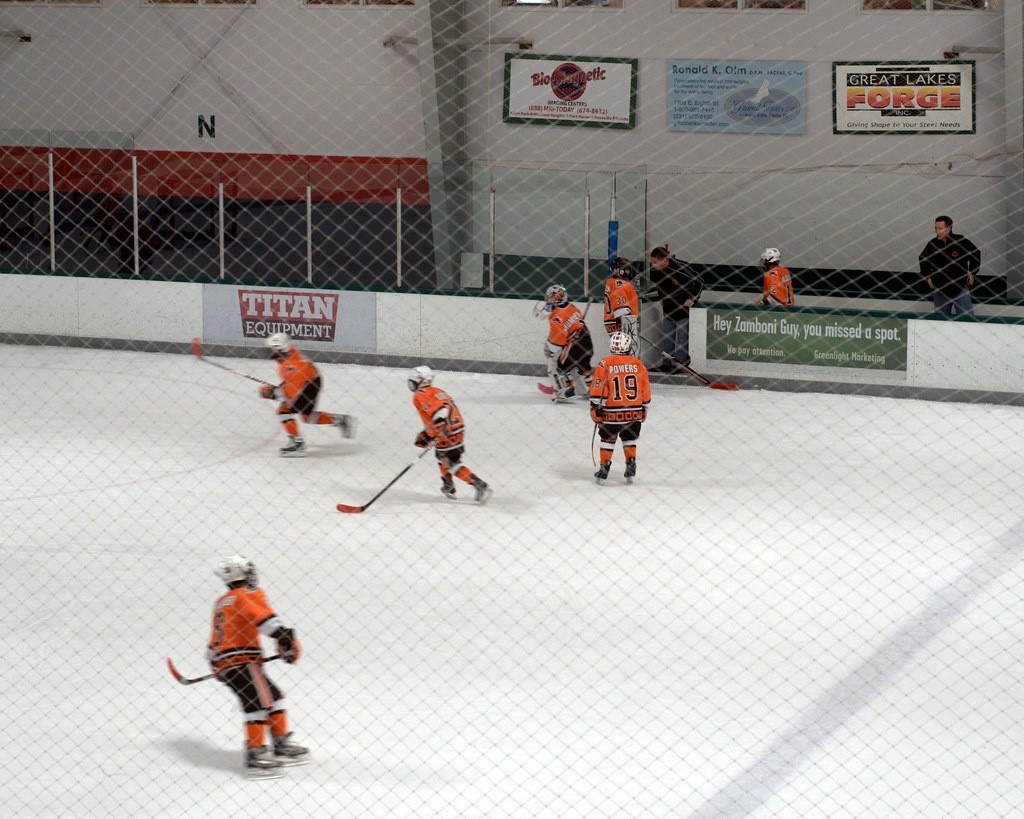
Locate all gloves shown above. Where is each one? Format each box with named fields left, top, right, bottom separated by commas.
left=432, top=418, right=448, bottom=436
left=213, top=667, right=226, bottom=682
left=544, top=343, right=553, bottom=359
left=590, top=401, right=603, bottom=423
left=753, top=300, right=764, bottom=306
left=642, top=404, right=649, bottom=422
left=277, top=629, right=301, bottom=664
left=259, top=386, right=279, bottom=400
left=414, top=429, right=434, bottom=448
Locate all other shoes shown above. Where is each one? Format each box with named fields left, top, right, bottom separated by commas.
left=671, top=368, right=684, bottom=374
left=650, top=364, right=672, bottom=372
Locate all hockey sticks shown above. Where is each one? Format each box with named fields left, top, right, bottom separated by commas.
left=638, top=334, right=740, bottom=391
left=336, top=438, right=440, bottom=513
left=591, top=368, right=609, bottom=468
left=536, top=296, right=593, bottom=395
left=192, top=337, right=277, bottom=388
left=166, top=653, right=282, bottom=686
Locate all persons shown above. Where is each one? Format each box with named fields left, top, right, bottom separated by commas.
left=756, top=248, right=794, bottom=306
left=919, top=216, right=981, bottom=315
left=408, top=366, right=493, bottom=505
left=603, top=257, right=640, bottom=358
left=205, top=555, right=309, bottom=781
left=642, top=247, right=703, bottom=374
left=589, top=333, right=651, bottom=485
left=260, top=333, right=357, bottom=457
left=544, top=285, right=595, bottom=400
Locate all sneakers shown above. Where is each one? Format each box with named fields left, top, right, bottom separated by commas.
left=555, top=379, right=576, bottom=399
left=244, top=745, right=287, bottom=779
left=440, top=477, right=457, bottom=499
left=271, top=731, right=313, bottom=767
left=624, top=457, right=636, bottom=483
left=467, top=473, right=494, bottom=504
left=593, top=461, right=612, bottom=486
left=330, top=414, right=360, bottom=439
left=585, top=381, right=591, bottom=398
left=278, top=435, right=308, bottom=457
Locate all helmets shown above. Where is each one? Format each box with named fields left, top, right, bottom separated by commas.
left=545, top=285, right=568, bottom=306
left=217, top=555, right=259, bottom=588
left=610, top=257, right=636, bottom=281
left=407, top=366, right=434, bottom=392
left=608, top=331, right=632, bottom=355
left=760, top=248, right=781, bottom=268
left=269, top=333, right=292, bottom=354
